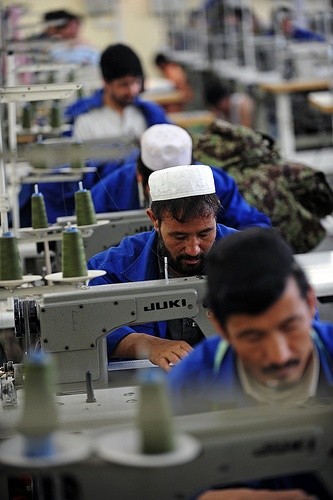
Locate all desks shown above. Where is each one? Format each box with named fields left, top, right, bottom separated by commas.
left=0, top=51, right=333, bottom=442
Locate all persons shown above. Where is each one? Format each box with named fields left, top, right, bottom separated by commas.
left=272, top=9, right=328, bottom=42
left=204, top=82, right=258, bottom=131
left=153, top=54, right=193, bottom=113
left=89, top=123, right=273, bottom=230
left=22, top=10, right=101, bottom=68
left=87, top=164, right=247, bottom=374
left=165, top=225, right=333, bottom=500
left=65, top=45, right=172, bottom=142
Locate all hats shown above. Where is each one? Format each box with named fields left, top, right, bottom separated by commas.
left=147, top=163, right=217, bottom=202
left=100, top=44, right=143, bottom=82
left=140, top=124, right=192, bottom=171
left=206, top=226, right=295, bottom=301
